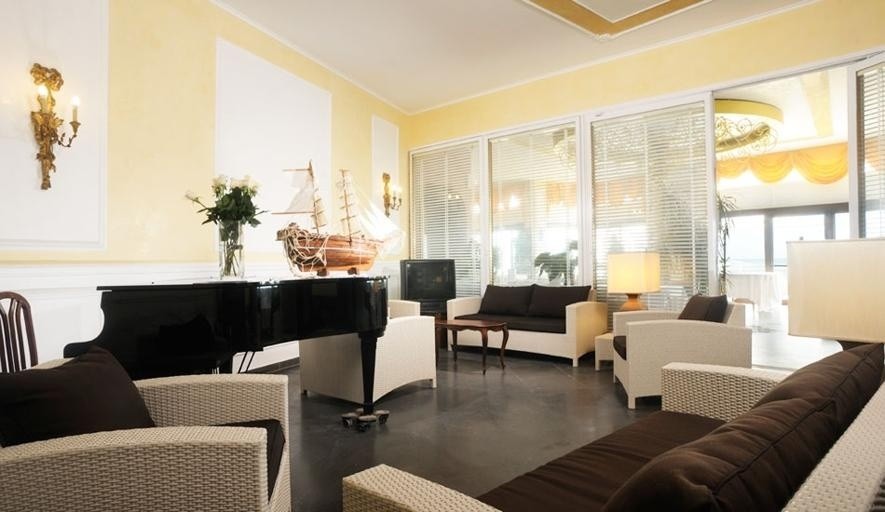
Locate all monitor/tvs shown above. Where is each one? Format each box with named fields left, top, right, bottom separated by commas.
left=400, top=259, right=456, bottom=302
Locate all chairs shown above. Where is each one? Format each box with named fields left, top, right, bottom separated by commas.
left=0, top=291, right=39, bottom=375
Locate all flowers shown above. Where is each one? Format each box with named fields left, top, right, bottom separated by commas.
left=183, top=173, right=272, bottom=228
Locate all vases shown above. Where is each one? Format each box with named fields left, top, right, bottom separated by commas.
left=217, top=223, right=246, bottom=280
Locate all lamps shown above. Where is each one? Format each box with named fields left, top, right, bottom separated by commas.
left=784, top=237, right=885, bottom=352
left=607, top=252, right=661, bottom=311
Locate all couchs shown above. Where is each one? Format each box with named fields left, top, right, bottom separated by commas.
left=612, top=302, right=753, bottom=410
left=340, top=361, right=885, bottom=512
left=446, top=289, right=607, bottom=368
left=299, top=299, right=436, bottom=406
left=0, top=358, right=293, bottom=512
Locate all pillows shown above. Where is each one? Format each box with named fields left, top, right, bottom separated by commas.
left=675, top=293, right=728, bottom=323
left=601, top=398, right=826, bottom=511
left=478, top=285, right=534, bottom=316
left=0, top=344, right=153, bottom=449
left=528, top=284, right=592, bottom=317
left=748, top=342, right=885, bottom=452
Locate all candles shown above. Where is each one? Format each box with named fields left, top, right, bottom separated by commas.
left=392, top=185, right=397, bottom=196
left=71, top=95, right=80, bottom=121
left=397, top=186, right=402, bottom=198
left=37, top=85, right=49, bottom=113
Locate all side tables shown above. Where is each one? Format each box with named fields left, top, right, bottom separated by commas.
left=725, top=271, right=776, bottom=314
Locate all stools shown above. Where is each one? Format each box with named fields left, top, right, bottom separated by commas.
left=594, top=332, right=615, bottom=371
